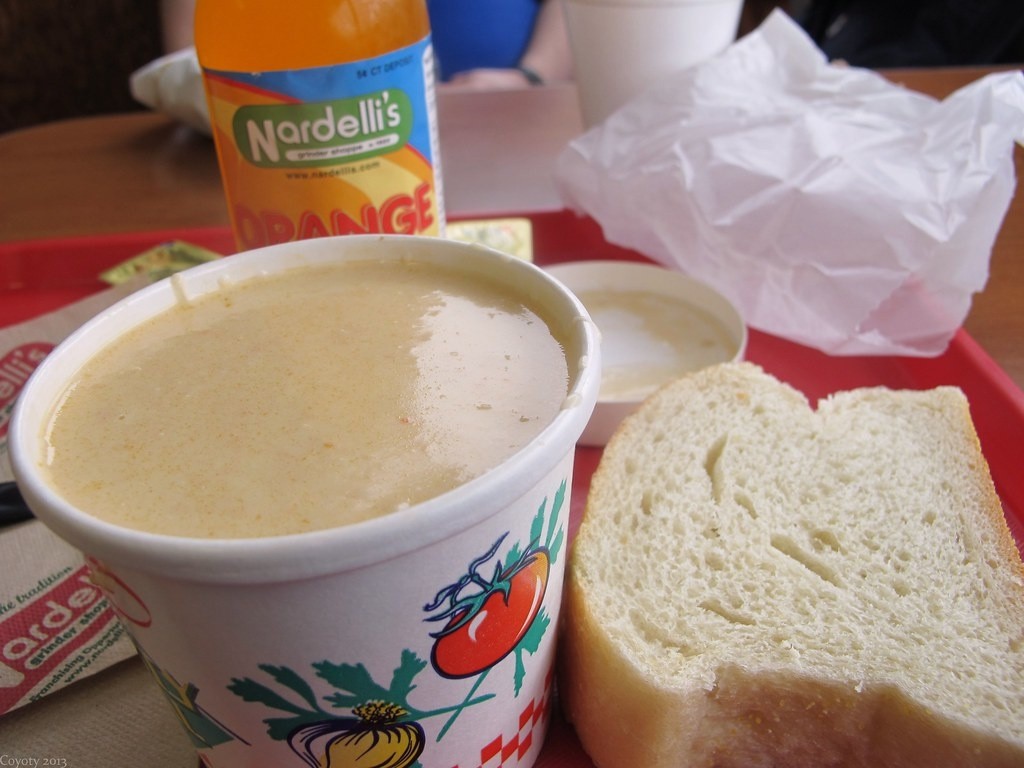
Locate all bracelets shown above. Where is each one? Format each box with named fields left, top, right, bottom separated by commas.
left=513, top=63, right=545, bottom=86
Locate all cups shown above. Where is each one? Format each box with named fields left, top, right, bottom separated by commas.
left=560, top=0, right=744, bottom=137
left=6, top=232, right=602, bottom=768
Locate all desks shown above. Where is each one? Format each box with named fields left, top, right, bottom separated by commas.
left=0, top=71, right=1024, bottom=768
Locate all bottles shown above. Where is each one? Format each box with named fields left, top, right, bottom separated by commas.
left=195, top=0, right=446, bottom=263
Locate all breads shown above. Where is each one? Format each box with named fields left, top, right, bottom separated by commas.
left=559, top=361, right=1024, bottom=768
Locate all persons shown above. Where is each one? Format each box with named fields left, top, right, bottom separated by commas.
left=160, top=0, right=572, bottom=93
